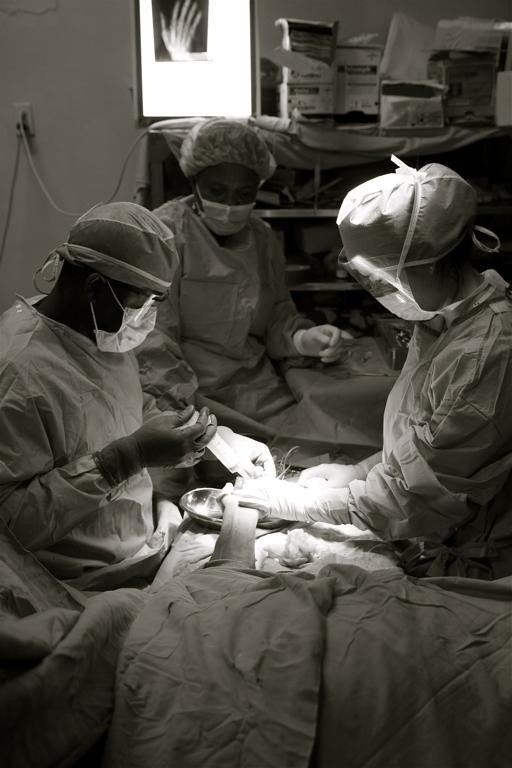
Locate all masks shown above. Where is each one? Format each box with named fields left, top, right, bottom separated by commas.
left=88, top=280, right=158, bottom=354
left=194, top=184, right=257, bottom=236
left=374, top=258, right=436, bottom=322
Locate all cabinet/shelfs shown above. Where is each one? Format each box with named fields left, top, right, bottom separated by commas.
left=144, top=125, right=511, bottom=294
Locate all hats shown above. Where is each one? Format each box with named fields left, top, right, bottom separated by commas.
left=68, top=201, right=180, bottom=293
left=336, top=162, right=478, bottom=264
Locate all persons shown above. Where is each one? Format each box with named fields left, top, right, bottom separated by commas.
left=132, top=115, right=363, bottom=469
left=157, top=0, right=205, bottom=63
left=207, top=458, right=283, bottom=573
left=225, top=151, right=510, bottom=601
left=1, top=198, right=278, bottom=593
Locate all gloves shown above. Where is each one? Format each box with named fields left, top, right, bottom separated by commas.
left=293, top=324, right=355, bottom=363
left=219, top=427, right=366, bottom=526
left=92, top=404, right=218, bottom=489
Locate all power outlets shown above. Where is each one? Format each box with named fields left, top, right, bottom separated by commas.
left=10, top=101, right=36, bottom=138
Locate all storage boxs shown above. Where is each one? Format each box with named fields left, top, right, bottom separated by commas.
left=275, top=17, right=511, bottom=132
